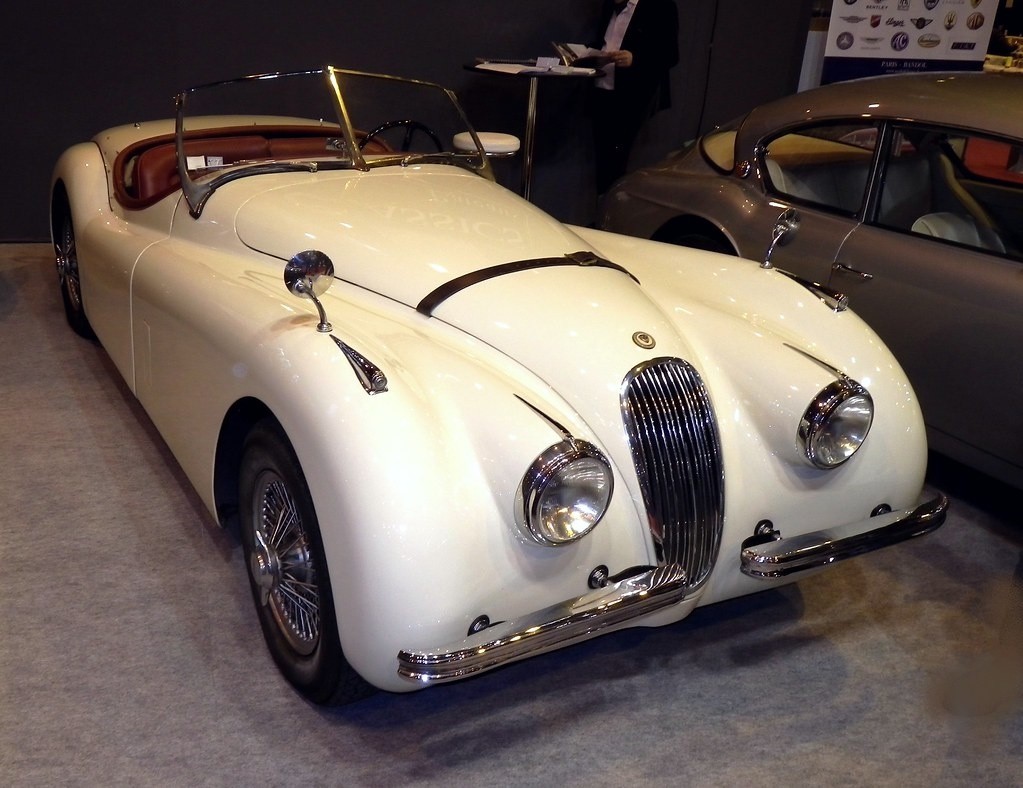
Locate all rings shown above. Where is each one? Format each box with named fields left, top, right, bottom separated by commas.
left=619, top=60, right=621, bottom=63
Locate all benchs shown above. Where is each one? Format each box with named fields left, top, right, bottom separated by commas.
left=781, top=156, right=932, bottom=229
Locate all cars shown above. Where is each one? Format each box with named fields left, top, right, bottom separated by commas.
left=598, top=70, right=1023, bottom=494
left=47, top=63, right=951, bottom=706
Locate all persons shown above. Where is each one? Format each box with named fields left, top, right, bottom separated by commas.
left=573, top=0, right=679, bottom=207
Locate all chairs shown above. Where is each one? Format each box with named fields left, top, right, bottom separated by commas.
left=912, top=212, right=1006, bottom=253
left=268, top=137, right=388, bottom=158
left=134, top=136, right=269, bottom=199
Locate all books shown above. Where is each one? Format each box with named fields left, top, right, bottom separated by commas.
left=552, top=42, right=613, bottom=69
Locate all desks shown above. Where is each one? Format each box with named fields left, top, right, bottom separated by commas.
left=463, top=61, right=607, bottom=201
